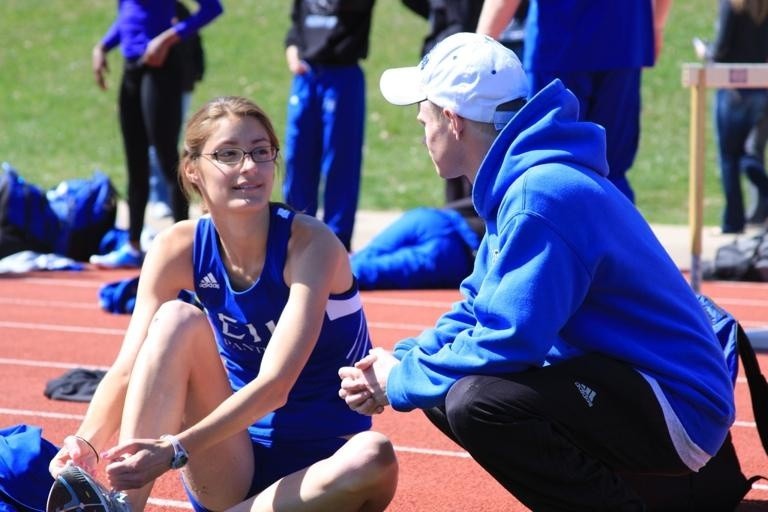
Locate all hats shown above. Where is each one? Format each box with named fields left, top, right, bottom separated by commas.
left=379, top=32, right=530, bottom=124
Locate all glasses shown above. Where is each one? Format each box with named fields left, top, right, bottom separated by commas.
left=197, top=145, right=280, bottom=166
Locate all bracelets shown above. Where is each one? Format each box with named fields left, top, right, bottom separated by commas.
left=76, top=435, right=99, bottom=464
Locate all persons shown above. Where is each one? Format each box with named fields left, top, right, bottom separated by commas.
left=47, top=95, right=398, bottom=512
left=397, top=0, right=527, bottom=201
left=85, top=0, right=225, bottom=270
left=336, top=27, right=737, bottom=511
left=711, top=0, right=767, bottom=234
left=281, top=1, right=374, bottom=250
left=475, top=0, right=675, bottom=206
left=349, top=194, right=485, bottom=289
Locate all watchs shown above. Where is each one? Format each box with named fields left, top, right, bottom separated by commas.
left=161, top=433, right=187, bottom=471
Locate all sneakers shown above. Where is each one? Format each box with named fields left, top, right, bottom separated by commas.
left=89, top=243, right=146, bottom=270
left=46, top=464, right=132, bottom=512
